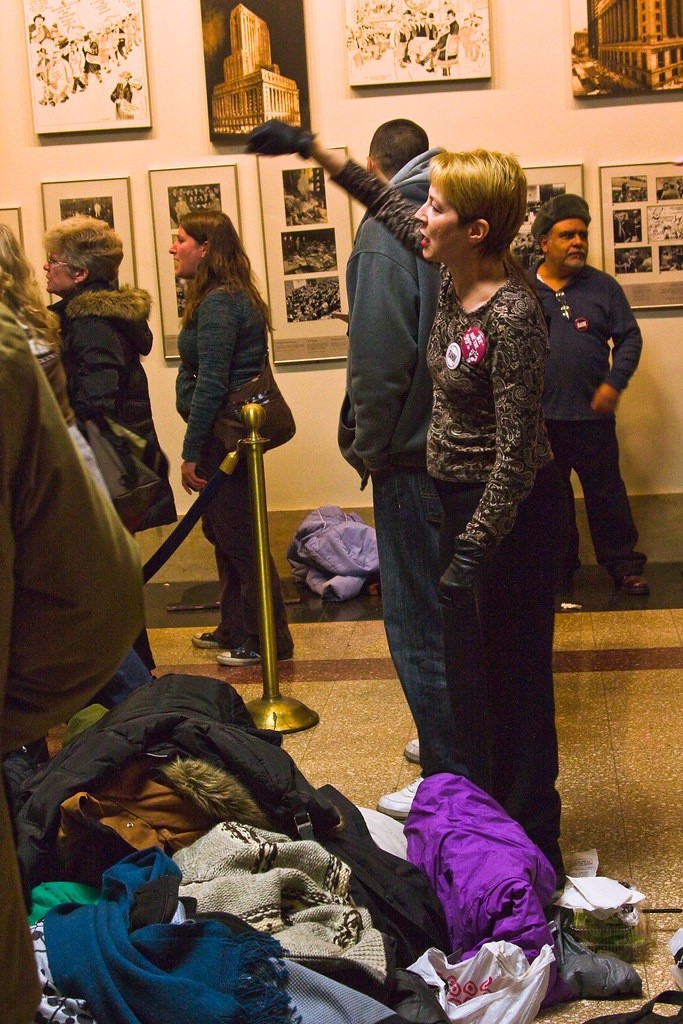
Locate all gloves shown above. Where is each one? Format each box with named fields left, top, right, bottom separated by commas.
left=437, top=538, right=484, bottom=607
left=246, top=119, right=315, bottom=161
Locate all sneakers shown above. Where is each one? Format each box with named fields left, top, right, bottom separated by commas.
left=404, top=739, right=421, bottom=763
left=376, top=776, right=426, bottom=819
left=191, top=631, right=227, bottom=648
left=216, top=645, right=262, bottom=666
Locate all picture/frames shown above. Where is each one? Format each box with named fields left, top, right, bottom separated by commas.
left=0, top=207, right=24, bottom=250
left=509, top=164, right=585, bottom=269
left=599, top=161, right=683, bottom=313
left=22, top=1, right=152, bottom=134
left=147, top=164, right=241, bottom=360
left=256, top=146, right=348, bottom=364
left=38, top=177, right=137, bottom=304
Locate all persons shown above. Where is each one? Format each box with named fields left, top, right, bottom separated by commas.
left=236, top=116, right=652, bottom=891
left=60, top=198, right=114, bottom=227
left=168, top=185, right=221, bottom=223
left=612, top=178, right=683, bottom=273
left=168, top=209, right=297, bottom=667
left=27, top=0, right=148, bottom=122
left=346, top=1, right=488, bottom=74
left=0, top=213, right=180, bottom=833
left=590, top=61, right=683, bottom=92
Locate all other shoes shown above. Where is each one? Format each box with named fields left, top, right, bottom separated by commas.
left=620, top=574, right=649, bottom=595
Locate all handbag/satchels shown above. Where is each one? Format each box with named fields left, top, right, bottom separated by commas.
left=200, top=363, right=296, bottom=456
left=405, top=940, right=556, bottom=1024
left=580, top=990, right=683, bottom=1024
left=72, top=401, right=163, bottom=523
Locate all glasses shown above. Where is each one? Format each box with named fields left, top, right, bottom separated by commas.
left=47, top=257, right=70, bottom=267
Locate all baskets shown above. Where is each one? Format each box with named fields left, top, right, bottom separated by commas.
left=569, top=877, right=639, bottom=963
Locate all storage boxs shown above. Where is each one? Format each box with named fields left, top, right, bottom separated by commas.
left=568, top=880, right=647, bottom=961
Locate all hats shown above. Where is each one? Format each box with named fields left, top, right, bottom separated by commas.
left=531, top=193, right=591, bottom=238
